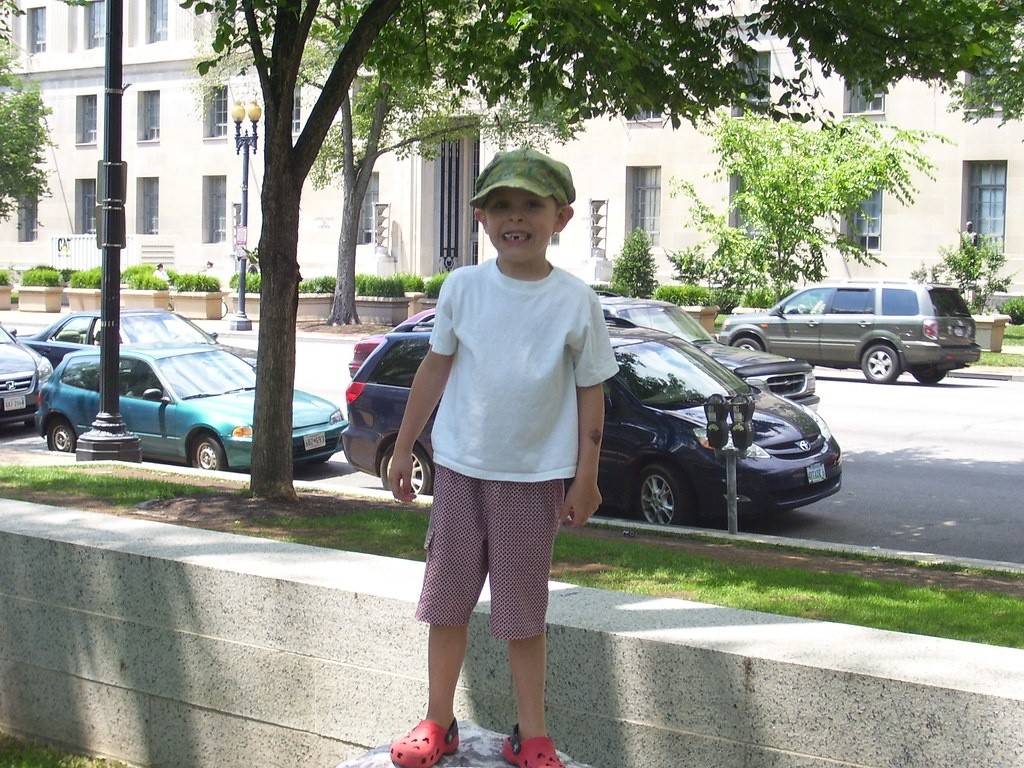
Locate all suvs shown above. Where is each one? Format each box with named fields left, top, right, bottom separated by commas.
left=717, top=280, right=981, bottom=386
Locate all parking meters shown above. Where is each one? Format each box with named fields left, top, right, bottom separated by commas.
left=703, top=392, right=757, bottom=536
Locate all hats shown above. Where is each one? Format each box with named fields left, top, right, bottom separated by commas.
left=467, top=147, right=575, bottom=209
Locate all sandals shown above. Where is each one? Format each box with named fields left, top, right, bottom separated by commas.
left=504, top=722, right=567, bottom=768
left=390, top=716, right=459, bottom=768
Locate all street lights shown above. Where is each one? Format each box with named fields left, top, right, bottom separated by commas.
left=229, top=98, right=263, bottom=330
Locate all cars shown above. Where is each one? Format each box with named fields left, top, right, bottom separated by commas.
left=343, top=303, right=437, bottom=381
left=1, top=320, right=55, bottom=430
left=598, top=292, right=821, bottom=409
left=29, top=340, right=349, bottom=473
left=336, top=310, right=842, bottom=532
left=13, top=305, right=257, bottom=374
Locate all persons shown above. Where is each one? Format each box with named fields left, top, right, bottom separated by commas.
left=203, top=261, right=219, bottom=278
left=154, top=263, right=169, bottom=280
left=385, top=148, right=620, bottom=768
